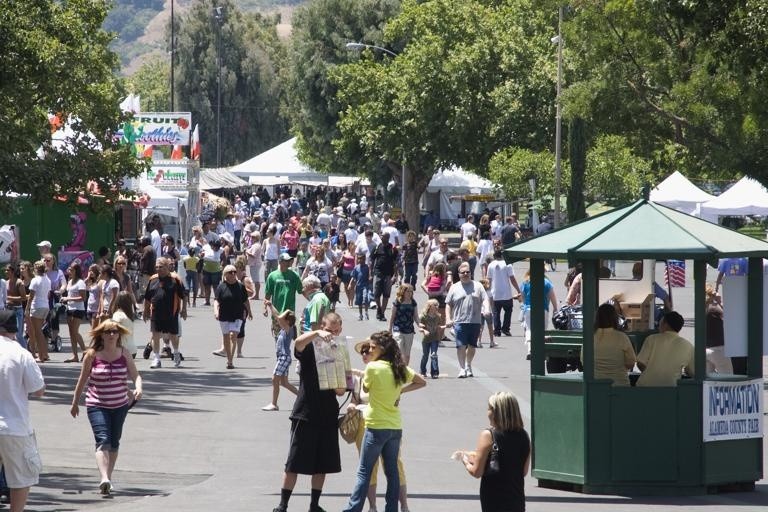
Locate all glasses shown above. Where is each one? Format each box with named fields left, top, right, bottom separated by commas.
left=43, top=260, right=50, bottom=263
left=459, top=271, right=471, bottom=275
left=102, top=329, right=120, bottom=335
left=227, top=271, right=236, bottom=274
left=117, top=263, right=125, bottom=265
left=359, top=350, right=369, bottom=355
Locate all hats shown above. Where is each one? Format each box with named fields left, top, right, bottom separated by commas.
left=249, top=230, right=260, bottom=238
left=352, top=336, right=373, bottom=354
left=35, top=240, right=52, bottom=249
left=218, top=232, right=232, bottom=243
left=279, top=252, right=293, bottom=263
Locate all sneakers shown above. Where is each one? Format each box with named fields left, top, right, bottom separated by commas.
left=375, top=308, right=387, bottom=322
left=418, top=370, right=427, bottom=377
left=143, top=342, right=185, bottom=368
left=272, top=503, right=286, bottom=512
left=224, top=360, right=235, bottom=370
left=525, top=352, right=531, bottom=360
left=356, top=312, right=370, bottom=323
left=430, top=373, right=438, bottom=379
left=456, top=366, right=475, bottom=380
left=211, top=348, right=228, bottom=358
left=475, top=342, right=483, bottom=349
left=260, top=402, right=280, bottom=411
left=97, top=479, right=114, bottom=496
left=488, top=340, right=498, bottom=349
left=493, top=327, right=512, bottom=337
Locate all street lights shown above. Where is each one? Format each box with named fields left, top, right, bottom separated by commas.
left=345, top=41, right=408, bottom=222
left=213, top=6, right=225, bottom=168
left=544, top=8, right=567, bottom=228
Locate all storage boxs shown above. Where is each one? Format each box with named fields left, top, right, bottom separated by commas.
left=608, top=292, right=657, bottom=333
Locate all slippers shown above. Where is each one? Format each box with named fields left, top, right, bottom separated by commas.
left=32, top=355, right=80, bottom=363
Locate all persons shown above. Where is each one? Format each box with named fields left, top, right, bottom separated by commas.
left=342, top=331, right=426, bottom=512
left=0, top=464, right=10, bottom=503
left=272, top=312, right=350, bottom=512
left=0, top=310, right=47, bottom=512
left=70, top=318, right=142, bottom=496
left=347, top=337, right=411, bottom=512
left=461, top=391, right=532, bottom=512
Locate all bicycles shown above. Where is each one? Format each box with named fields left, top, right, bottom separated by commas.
left=546, top=257, right=558, bottom=271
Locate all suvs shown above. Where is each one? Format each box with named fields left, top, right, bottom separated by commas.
left=722, top=214, right=747, bottom=231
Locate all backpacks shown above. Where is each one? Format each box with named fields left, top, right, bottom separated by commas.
left=425, top=273, right=444, bottom=294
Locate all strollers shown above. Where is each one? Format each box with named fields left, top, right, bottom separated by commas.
left=23, top=291, right=65, bottom=354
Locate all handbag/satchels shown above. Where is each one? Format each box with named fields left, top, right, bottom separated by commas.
left=552, top=304, right=573, bottom=330
left=481, top=426, right=500, bottom=479
left=335, top=409, right=361, bottom=446
